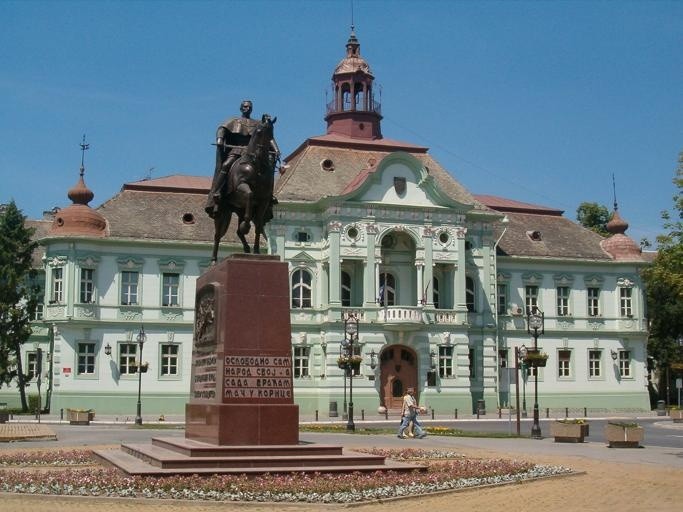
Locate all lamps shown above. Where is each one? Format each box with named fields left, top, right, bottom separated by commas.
left=611, top=349, right=617, bottom=360
left=104, top=343, right=111, bottom=355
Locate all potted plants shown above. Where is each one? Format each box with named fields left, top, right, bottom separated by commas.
left=669, top=407, right=683, bottom=423
left=336, top=355, right=362, bottom=371
left=527, top=353, right=549, bottom=367
left=67, top=408, right=92, bottom=424
left=605, top=422, right=644, bottom=448
left=550, top=418, right=589, bottom=443
left=129, top=361, right=138, bottom=373
left=140, top=362, right=148, bottom=372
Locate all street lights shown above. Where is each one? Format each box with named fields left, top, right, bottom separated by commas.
left=527, top=307, right=544, bottom=439
left=135, top=323, right=146, bottom=425
left=517, top=343, right=528, bottom=418
left=344, top=314, right=358, bottom=432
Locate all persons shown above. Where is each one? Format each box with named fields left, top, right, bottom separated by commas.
left=395, top=386, right=427, bottom=440
left=203, top=98, right=280, bottom=220
left=399, top=385, right=423, bottom=439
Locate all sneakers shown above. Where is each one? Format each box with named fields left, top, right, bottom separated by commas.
left=397, top=430, right=427, bottom=439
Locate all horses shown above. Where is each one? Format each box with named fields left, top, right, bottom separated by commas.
left=210, top=113, right=276, bottom=267
